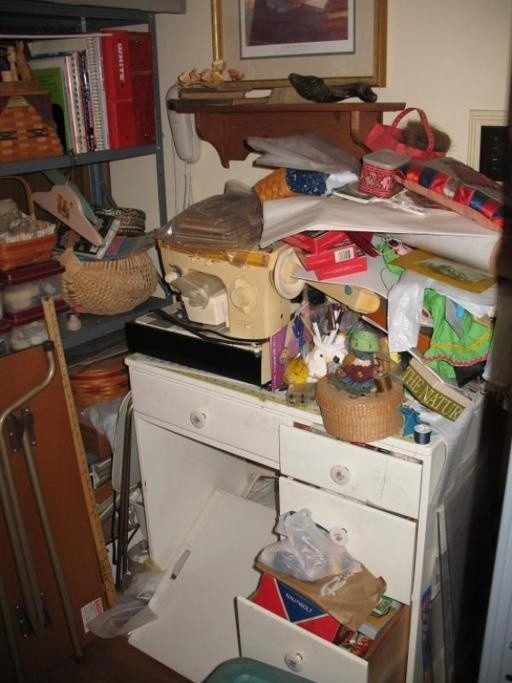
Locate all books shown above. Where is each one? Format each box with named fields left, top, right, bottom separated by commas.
left=30, top=27, right=156, bottom=156
left=257, top=574, right=343, bottom=643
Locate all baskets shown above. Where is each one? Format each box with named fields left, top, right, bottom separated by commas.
left=55, top=247, right=159, bottom=315
left=0, top=175, right=58, bottom=271
left=317, top=372, right=405, bottom=442
left=253, top=166, right=300, bottom=203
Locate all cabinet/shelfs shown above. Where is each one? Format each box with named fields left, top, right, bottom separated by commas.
left=122, top=352, right=485, bottom=683
left=1, top=296, right=124, bottom=681
left=1, top=0, right=184, bottom=368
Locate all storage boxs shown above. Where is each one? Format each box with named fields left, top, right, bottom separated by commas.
left=1, top=258, right=72, bottom=352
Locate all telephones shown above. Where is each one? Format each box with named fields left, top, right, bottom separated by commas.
left=166, top=84, right=201, bottom=163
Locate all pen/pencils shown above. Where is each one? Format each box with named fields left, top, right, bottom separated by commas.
left=299, top=310, right=344, bottom=344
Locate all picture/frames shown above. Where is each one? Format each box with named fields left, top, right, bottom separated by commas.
left=467, top=109, right=511, bottom=186
left=210, top=0, right=388, bottom=88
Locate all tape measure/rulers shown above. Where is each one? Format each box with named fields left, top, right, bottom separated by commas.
left=42, top=295, right=118, bottom=607
